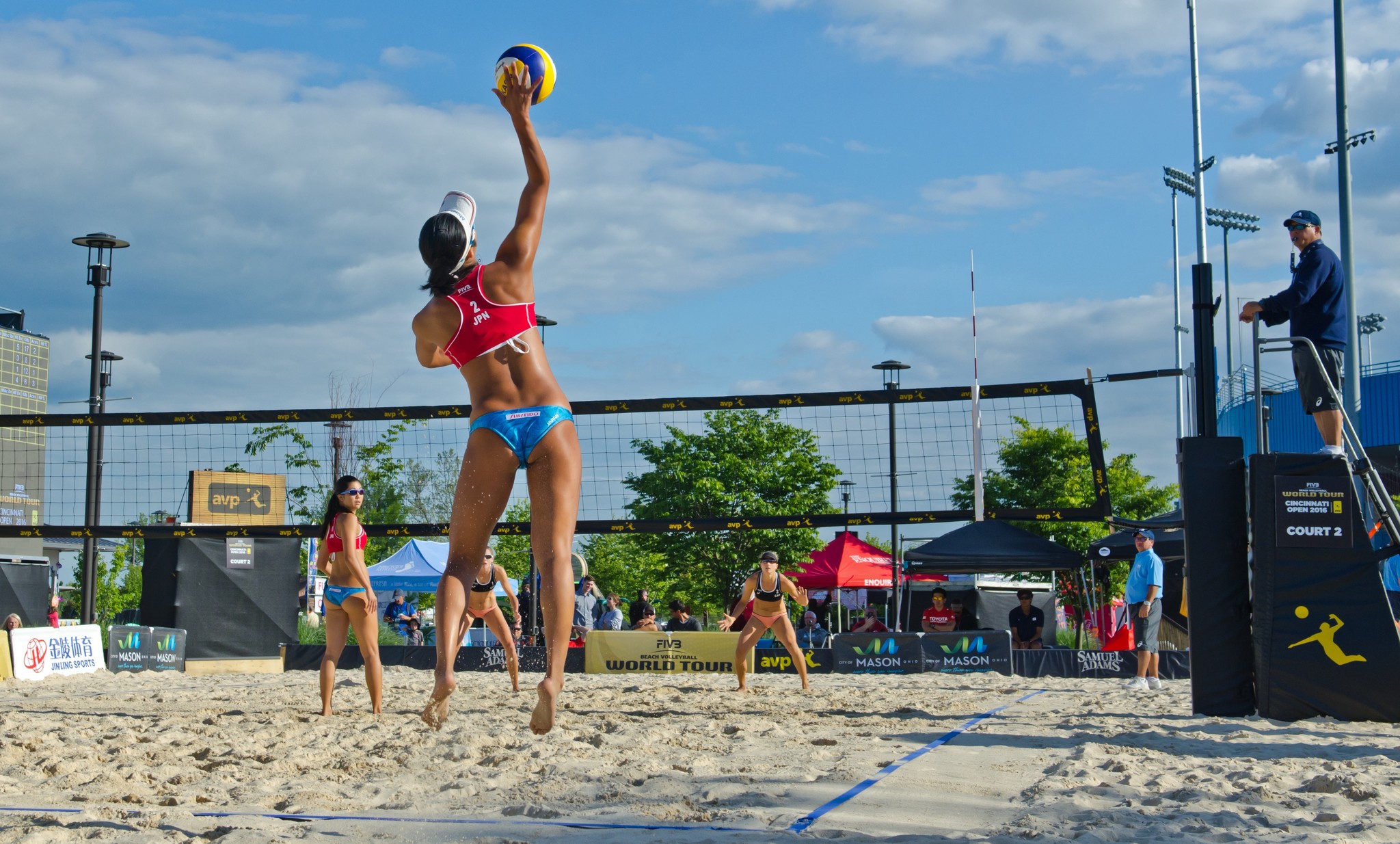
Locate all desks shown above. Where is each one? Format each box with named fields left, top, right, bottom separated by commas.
left=420, top=626, right=472, bottom=646
left=851, top=617, right=885, bottom=627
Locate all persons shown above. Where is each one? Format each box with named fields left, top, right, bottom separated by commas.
left=2, top=604, right=58, bottom=675
left=412, top=60, right=580, bottom=735
left=1239, top=209, right=1348, bottom=458
left=307, top=475, right=1164, bottom=718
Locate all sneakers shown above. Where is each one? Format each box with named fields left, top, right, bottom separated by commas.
left=1146, top=676, right=1162, bottom=689
left=1122, top=676, right=1150, bottom=691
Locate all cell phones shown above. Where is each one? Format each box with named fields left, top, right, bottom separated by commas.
left=868, top=612, right=873, bottom=619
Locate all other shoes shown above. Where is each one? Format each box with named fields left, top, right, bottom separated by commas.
left=1313, top=446, right=1345, bottom=455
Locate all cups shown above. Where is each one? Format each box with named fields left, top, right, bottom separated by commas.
left=398, top=613, right=403, bottom=619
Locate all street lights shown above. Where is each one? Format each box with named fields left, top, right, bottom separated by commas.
left=1356, top=312, right=1388, bottom=377
left=1203, top=206, right=1262, bottom=408
left=1324, top=129, right=1378, bottom=528
left=70, top=232, right=131, bottom=627
left=872, top=359, right=912, bottom=632
left=1162, top=165, right=1196, bottom=438
left=836, top=479, right=857, bottom=531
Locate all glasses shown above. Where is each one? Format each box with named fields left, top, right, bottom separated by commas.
left=933, top=596, right=944, bottom=601
left=484, top=555, right=494, bottom=559
left=760, top=557, right=776, bottom=564
left=645, top=595, right=649, bottom=597
left=511, top=627, right=521, bottom=631
left=1134, top=536, right=1149, bottom=542
left=339, top=489, right=365, bottom=496
left=670, top=611, right=676, bottom=614
left=1019, top=596, right=1031, bottom=600
left=1287, top=224, right=1317, bottom=232
left=607, top=597, right=612, bottom=600
left=644, top=613, right=654, bottom=616
left=955, top=611, right=960, bottom=614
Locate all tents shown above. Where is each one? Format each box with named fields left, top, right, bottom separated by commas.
left=366, top=538, right=518, bottom=647
left=777, top=505, right=1188, bottom=653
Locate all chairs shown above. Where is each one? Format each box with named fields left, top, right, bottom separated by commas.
left=755, top=639, right=776, bottom=648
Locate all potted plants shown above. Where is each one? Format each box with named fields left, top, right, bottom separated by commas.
left=166, top=514, right=175, bottom=523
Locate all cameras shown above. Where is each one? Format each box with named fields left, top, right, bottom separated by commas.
left=807, top=620, right=813, bottom=626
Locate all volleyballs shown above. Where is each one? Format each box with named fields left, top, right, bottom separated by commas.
left=494, top=43, right=557, bottom=106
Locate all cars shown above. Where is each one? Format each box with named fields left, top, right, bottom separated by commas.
left=659, top=621, right=668, bottom=627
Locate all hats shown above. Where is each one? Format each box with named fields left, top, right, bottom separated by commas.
left=761, top=552, right=779, bottom=563
left=643, top=605, right=656, bottom=615
left=1284, top=210, right=1321, bottom=228
left=406, top=617, right=421, bottom=630
left=1133, top=529, right=1154, bottom=540
left=392, top=588, right=404, bottom=600
left=438, top=191, right=477, bottom=277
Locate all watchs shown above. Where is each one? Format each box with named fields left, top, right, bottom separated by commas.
left=1143, top=600, right=1151, bottom=606
